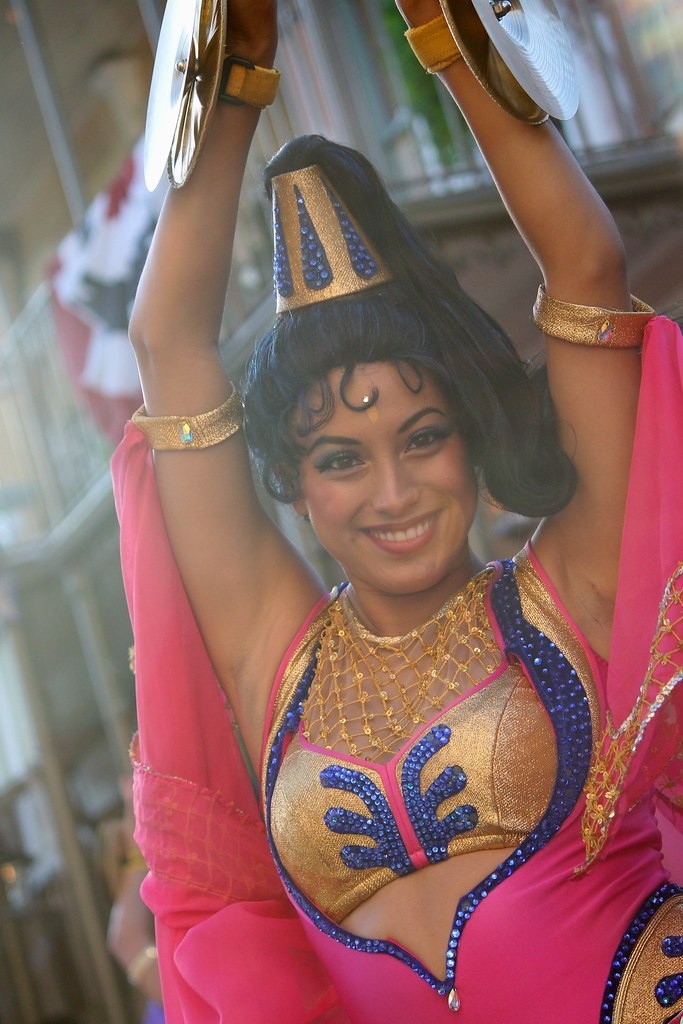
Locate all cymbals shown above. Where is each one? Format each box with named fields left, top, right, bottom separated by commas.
left=438, top=0, right=585, bottom=127
left=139, top=0, right=228, bottom=195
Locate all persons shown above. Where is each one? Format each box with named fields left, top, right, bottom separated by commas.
left=122, top=0, right=683, bottom=1024
left=103, top=838, right=171, bottom=1010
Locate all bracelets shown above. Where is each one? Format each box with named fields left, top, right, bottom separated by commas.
left=124, top=943, right=159, bottom=990
